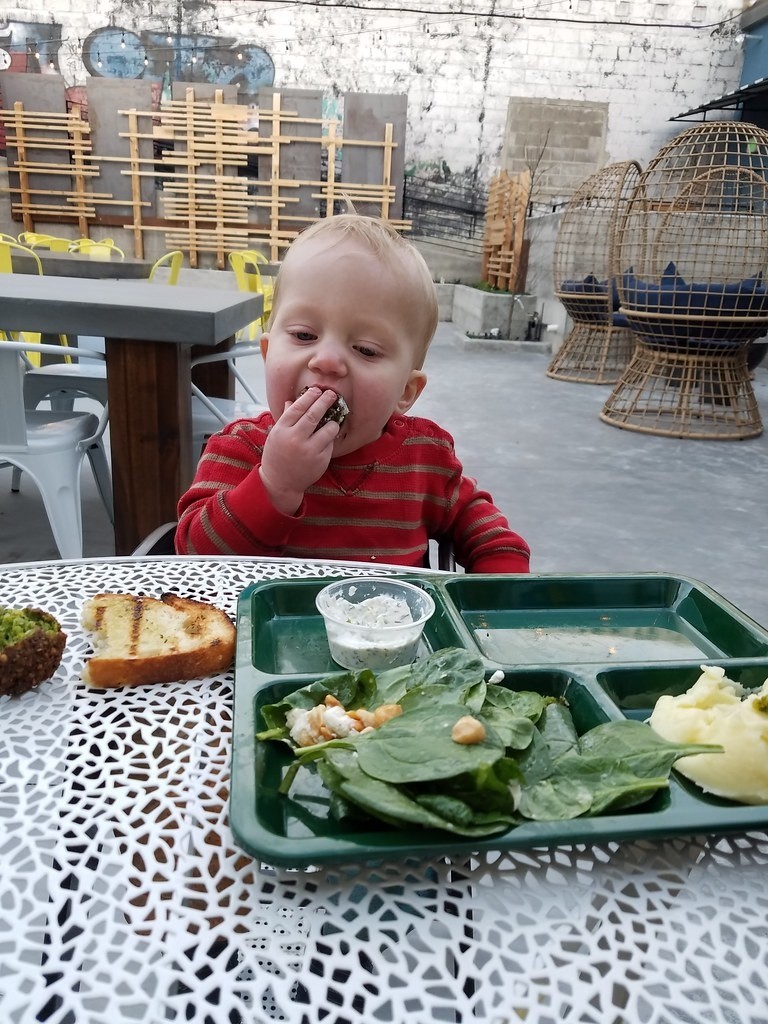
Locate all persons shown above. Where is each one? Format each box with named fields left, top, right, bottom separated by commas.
left=174, top=215, right=531, bottom=574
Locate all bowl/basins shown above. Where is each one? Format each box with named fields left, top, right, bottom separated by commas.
left=315, top=577, right=435, bottom=671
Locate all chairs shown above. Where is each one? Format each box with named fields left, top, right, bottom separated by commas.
left=228, top=250, right=275, bottom=343
left=0, top=338, right=114, bottom=560
left=0, top=231, right=125, bottom=257
left=599, top=121, right=768, bottom=441
left=191, top=341, right=268, bottom=481
left=545, top=160, right=646, bottom=385
left=0, top=241, right=72, bottom=368
left=148, top=250, right=184, bottom=285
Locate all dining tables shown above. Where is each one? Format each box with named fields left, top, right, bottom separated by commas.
left=0, top=560, right=768, bottom=1024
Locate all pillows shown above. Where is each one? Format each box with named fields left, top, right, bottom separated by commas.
left=632, top=262, right=768, bottom=356
left=561, top=272, right=609, bottom=320
left=599, top=266, right=634, bottom=312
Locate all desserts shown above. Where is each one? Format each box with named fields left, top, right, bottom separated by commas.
left=646, top=663, right=768, bottom=806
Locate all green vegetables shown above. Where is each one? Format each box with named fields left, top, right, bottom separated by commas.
left=256, top=643, right=726, bottom=842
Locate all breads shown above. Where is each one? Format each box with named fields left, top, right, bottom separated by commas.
left=79, top=591, right=237, bottom=688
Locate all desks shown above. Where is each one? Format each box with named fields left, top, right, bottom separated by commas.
left=0, top=272, right=263, bottom=556
